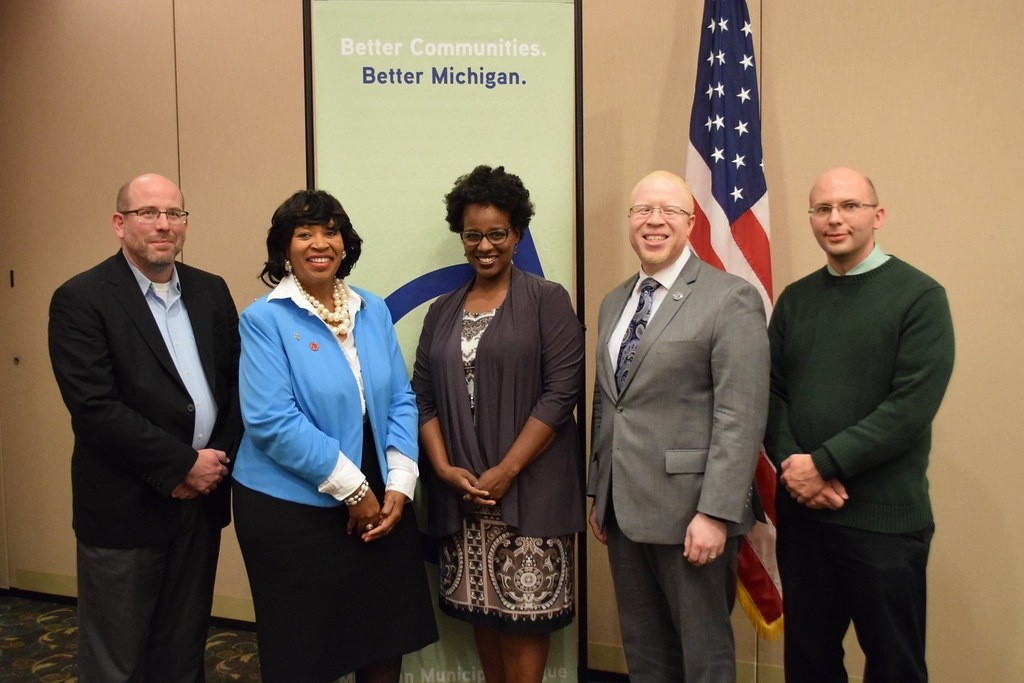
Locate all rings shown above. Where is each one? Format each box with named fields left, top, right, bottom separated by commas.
left=709, top=556, right=716, bottom=559
left=366, top=523, right=374, bottom=531
left=372, top=535, right=376, bottom=540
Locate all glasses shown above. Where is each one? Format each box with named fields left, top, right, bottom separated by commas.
left=119, top=209, right=188, bottom=226
left=809, top=202, right=878, bottom=216
left=461, top=227, right=514, bottom=246
left=629, top=204, right=691, bottom=217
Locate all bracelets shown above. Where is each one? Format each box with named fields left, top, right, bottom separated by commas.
left=343, top=477, right=369, bottom=505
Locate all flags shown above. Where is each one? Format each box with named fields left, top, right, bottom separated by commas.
left=679, top=1, right=773, bottom=635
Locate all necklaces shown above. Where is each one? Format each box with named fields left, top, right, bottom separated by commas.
left=292, top=271, right=353, bottom=336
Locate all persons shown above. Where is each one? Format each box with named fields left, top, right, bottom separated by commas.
left=765, top=167, right=956, bottom=683
left=587, top=171, right=771, bottom=683
left=411, top=166, right=587, bottom=683
left=231, top=191, right=439, bottom=683
left=47, top=174, right=242, bottom=683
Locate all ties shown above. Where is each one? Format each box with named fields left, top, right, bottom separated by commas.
left=615, top=277, right=660, bottom=395
